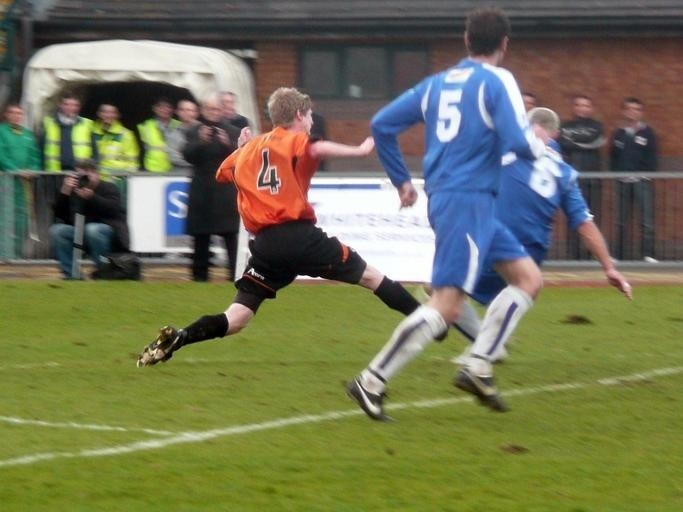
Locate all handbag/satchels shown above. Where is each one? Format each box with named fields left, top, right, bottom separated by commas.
left=91, top=251, right=142, bottom=282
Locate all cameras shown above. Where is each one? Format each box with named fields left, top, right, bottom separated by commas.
left=75, top=173, right=90, bottom=189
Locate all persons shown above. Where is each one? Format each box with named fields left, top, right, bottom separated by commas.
left=309, top=114, right=325, bottom=173
left=48, top=159, right=129, bottom=282
left=340, top=4, right=551, bottom=423
left=607, top=95, right=661, bottom=263
left=37, top=90, right=253, bottom=223
left=554, top=94, right=609, bottom=261
left=182, top=95, right=250, bottom=283
left=133, top=84, right=449, bottom=370
left=519, top=90, right=537, bottom=113
left=1, top=105, right=41, bottom=258
left=419, top=106, right=634, bottom=367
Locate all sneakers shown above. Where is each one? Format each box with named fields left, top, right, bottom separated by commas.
left=450, top=365, right=511, bottom=413
left=136, top=324, right=184, bottom=370
left=343, top=377, right=398, bottom=425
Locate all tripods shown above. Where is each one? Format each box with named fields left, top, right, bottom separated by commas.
left=70, top=198, right=86, bottom=280
